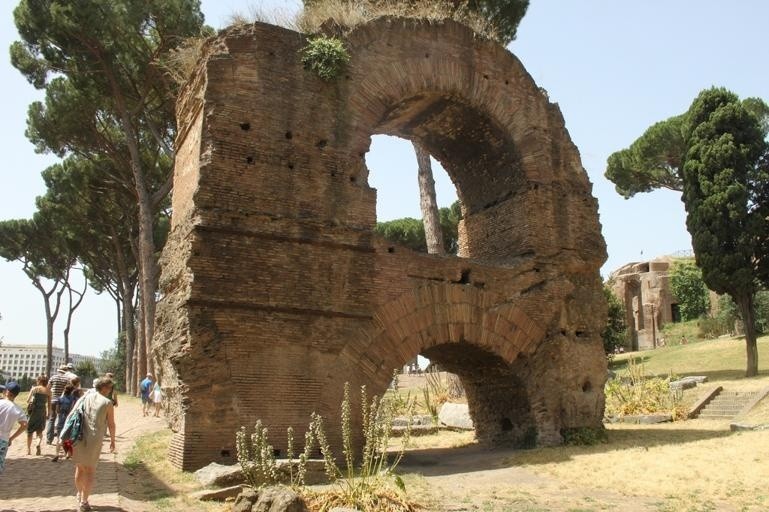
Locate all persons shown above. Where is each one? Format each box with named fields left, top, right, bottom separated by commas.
left=25, top=374, right=52, bottom=455
left=656, top=338, right=659, bottom=347
left=0, top=381, right=27, bottom=473
left=140, top=373, right=154, bottom=417
left=152, top=380, right=162, bottom=418
left=402, top=362, right=421, bottom=376
left=46, top=362, right=84, bottom=463
left=661, top=337, right=665, bottom=347
left=680, top=336, right=688, bottom=344
left=65, top=378, right=116, bottom=511
left=614, top=343, right=625, bottom=354
left=104, top=373, right=118, bottom=437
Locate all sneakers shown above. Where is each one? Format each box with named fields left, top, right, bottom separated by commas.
left=51, top=456, right=59, bottom=462
left=36, top=444, right=41, bottom=456
left=77, top=494, right=91, bottom=512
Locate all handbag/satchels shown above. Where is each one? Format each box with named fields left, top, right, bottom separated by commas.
left=28, top=402, right=33, bottom=414
left=60, top=401, right=82, bottom=445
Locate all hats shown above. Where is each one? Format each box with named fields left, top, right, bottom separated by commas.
left=5, top=381, right=21, bottom=395
left=66, top=362, right=76, bottom=371
left=57, top=365, right=67, bottom=374
